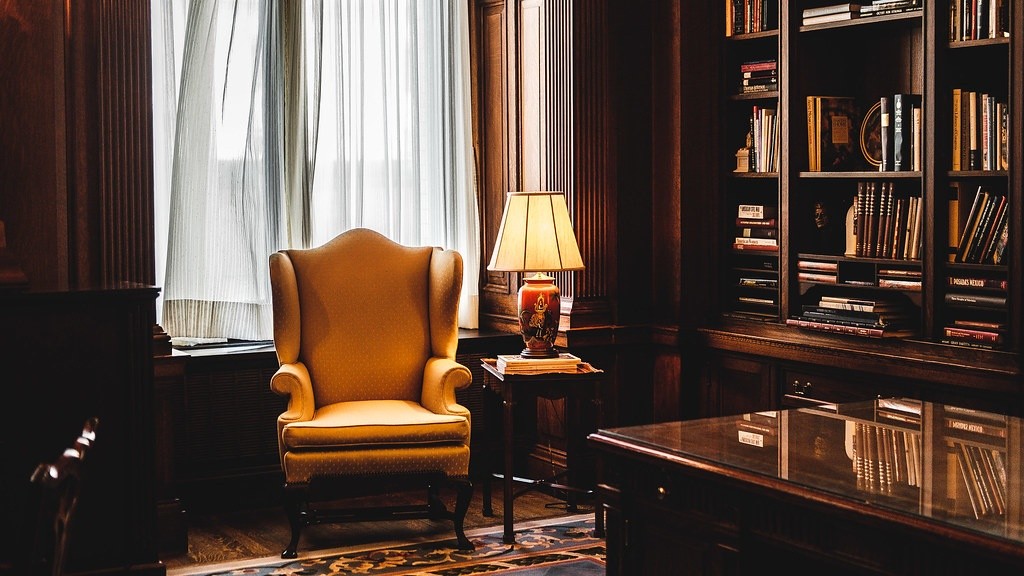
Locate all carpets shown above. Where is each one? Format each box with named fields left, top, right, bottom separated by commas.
left=165, top=511, right=608, bottom=576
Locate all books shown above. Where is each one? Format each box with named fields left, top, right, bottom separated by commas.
left=736, top=411, right=778, bottom=448
left=750, top=105, right=779, bottom=172
left=953, top=89, right=1008, bottom=171
left=940, top=277, right=1008, bottom=349
left=856, top=181, right=923, bottom=259
left=726, top=0, right=767, bottom=36
left=946, top=441, right=1007, bottom=520
left=733, top=218, right=779, bottom=252
left=739, top=297, right=774, bottom=303
left=739, top=278, right=777, bottom=288
left=741, top=59, right=777, bottom=93
left=880, top=94, right=921, bottom=172
left=786, top=260, right=921, bottom=337
left=803, top=0, right=923, bottom=25
left=497, top=355, right=581, bottom=374
left=948, top=181, right=1008, bottom=265
left=807, top=96, right=861, bottom=171
left=948, top=0, right=1004, bottom=42
left=854, top=421, right=921, bottom=495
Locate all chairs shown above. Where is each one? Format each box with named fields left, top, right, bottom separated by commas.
left=269, top=227, right=475, bottom=559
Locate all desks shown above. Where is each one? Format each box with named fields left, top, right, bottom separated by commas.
left=0, top=274, right=189, bottom=569
left=480, top=358, right=605, bottom=545
left=586, top=394, right=1023, bottom=576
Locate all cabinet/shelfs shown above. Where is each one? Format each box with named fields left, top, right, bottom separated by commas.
left=702, top=1, right=1023, bottom=418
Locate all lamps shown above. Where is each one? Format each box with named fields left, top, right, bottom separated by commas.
left=487, top=192, right=585, bottom=359
left=29, top=415, right=100, bottom=576
